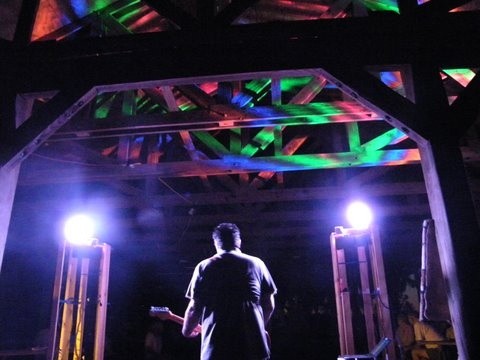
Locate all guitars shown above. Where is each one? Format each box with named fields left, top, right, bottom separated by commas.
left=149, top=304, right=271, bottom=357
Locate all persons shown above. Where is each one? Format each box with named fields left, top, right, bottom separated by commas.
left=181, top=223, right=276, bottom=359
